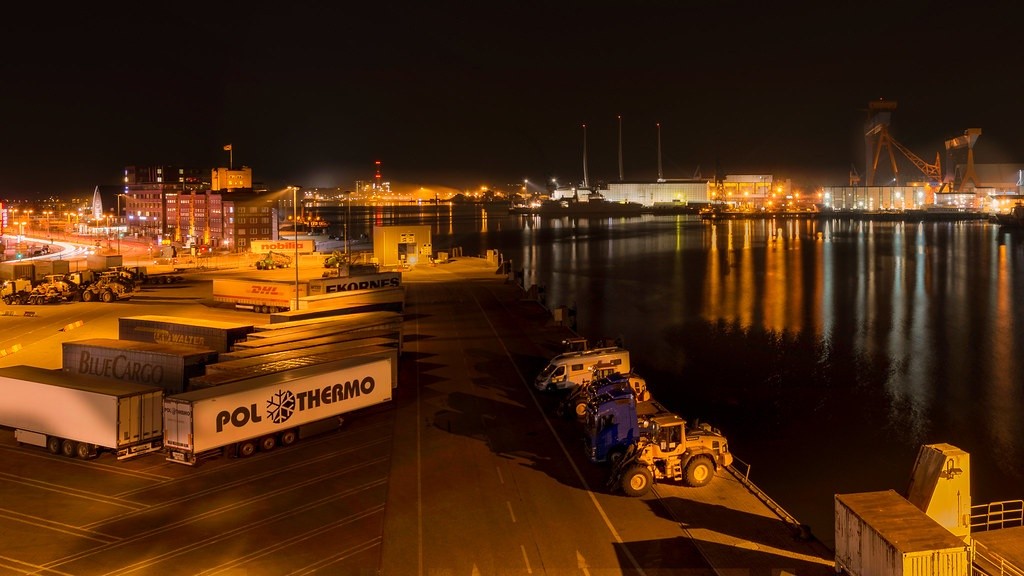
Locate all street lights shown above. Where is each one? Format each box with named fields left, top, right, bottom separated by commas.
left=103, top=215, right=113, bottom=229
left=64, top=212, right=83, bottom=233
left=114, top=194, right=123, bottom=255
left=618, top=116, right=623, bottom=180
left=9, top=209, right=17, bottom=223
left=524, top=179, right=528, bottom=205
left=23, top=210, right=33, bottom=229
left=288, top=186, right=302, bottom=310
left=657, top=123, right=662, bottom=179
left=44, top=211, right=53, bottom=233
left=582, top=124, right=587, bottom=186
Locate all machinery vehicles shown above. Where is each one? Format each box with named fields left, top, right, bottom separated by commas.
left=556, top=362, right=733, bottom=497
left=257, top=252, right=293, bottom=269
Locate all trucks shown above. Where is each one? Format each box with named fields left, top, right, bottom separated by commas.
left=1, top=266, right=185, bottom=305
left=534, top=346, right=630, bottom=399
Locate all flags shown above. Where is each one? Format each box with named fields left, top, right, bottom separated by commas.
left=224, top=145, right=231, bottom=150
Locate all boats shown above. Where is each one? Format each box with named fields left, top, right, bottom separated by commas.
left=541, top=183, right=642, bottom=215
left=995, top=203, right=1024, bottom=227
